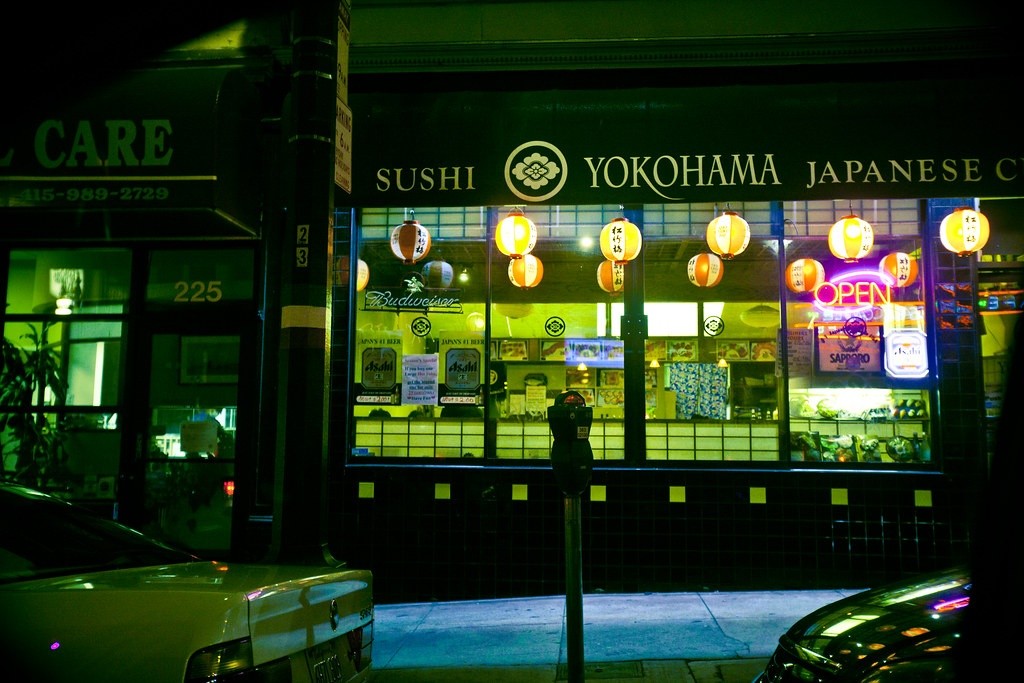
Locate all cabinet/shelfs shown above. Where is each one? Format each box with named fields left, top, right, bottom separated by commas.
left=731, top=361, right=775, bottom=422
left=789, top=388, right=930, bottom=462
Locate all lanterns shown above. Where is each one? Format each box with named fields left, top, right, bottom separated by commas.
left=596, top=261, right=625, bottom=296
left=706, top=211, right=750, bottom=259
left=494, top=208, right=537, bottom=259
left=422, top=256, right=453, bottom=288
left=508, top=253, right=544, bottom=290
left=390, top=220, right=431, bottom=264
left=940, top=205, right=990, bottom=257
left=687, top=251, right=724, bottom=289
left=878, top=250, right=919, bottom=288
left=599, top=217, right=643, bottom=265
left=828, top=214, right=874, bottom=262
left=356, top=258, right=370, bottom=291
left=785, top=256, right=825, bottom=293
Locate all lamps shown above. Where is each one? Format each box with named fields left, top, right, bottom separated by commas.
left=336, top=257, right=370, bottom=292
left=784, top=256, right=826, bottom=293
left=597, top=259, right=625, bottom=297
left=828, top=199, right=874, bottom=262
left=688, top=250, right=725, bottom=288
left=600, top=204, right=643, bottom=265
left=423, top=248, right=455, bottom=295
left=496, top=208, right=539, bottom=260
left=707, top=203, right=751, bottom=259
left=509, top=254, right=545, bottom=289
left=940, top=197, right=990, bottom=257
left=879, top=243, right=919, bottom=287
left=391, top=208, right=431, bottom=264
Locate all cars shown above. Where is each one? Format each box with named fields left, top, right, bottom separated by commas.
left=751, top=564, right=978, bottom=683
left=1, top=477, right=376, bottom=683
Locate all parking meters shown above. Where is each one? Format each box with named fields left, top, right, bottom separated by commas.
left=546, top=391, right=595, bottom=683
